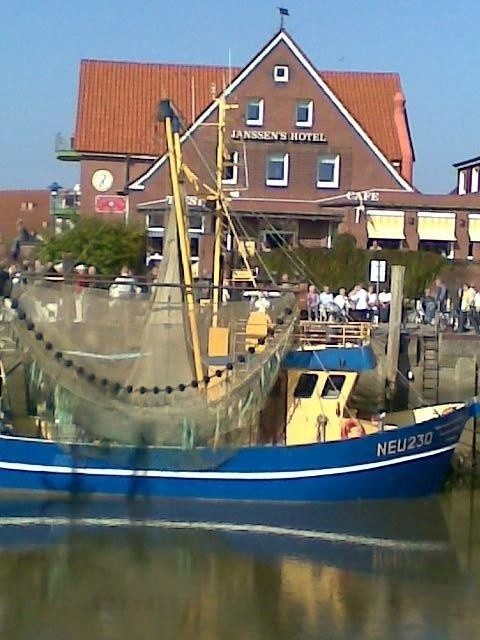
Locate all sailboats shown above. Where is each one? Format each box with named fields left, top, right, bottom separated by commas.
left=0, top=494, right=470, bottom=639
left=0, top=70, right=478, bottom=502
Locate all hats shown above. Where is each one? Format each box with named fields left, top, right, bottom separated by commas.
left=75, top=264, right=85, bottom=270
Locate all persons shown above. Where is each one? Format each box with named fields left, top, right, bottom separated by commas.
left=369, top=239, right=383, bottom=251
left=0, top=250, right=160, bottom=323
left=282, top=273, right=480, bottom=335
